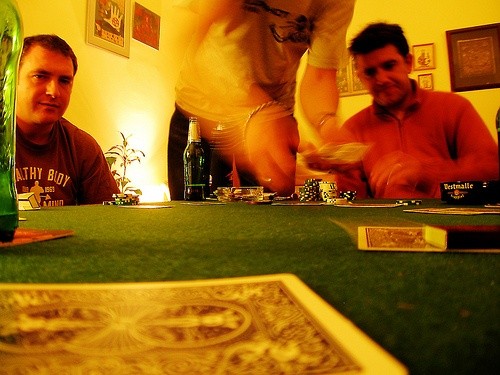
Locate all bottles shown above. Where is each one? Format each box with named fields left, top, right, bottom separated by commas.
left=0, top=0, right=23, bottom=243
left=183, top=116, right=208, bottom=201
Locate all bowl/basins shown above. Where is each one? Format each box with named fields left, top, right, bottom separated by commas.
left=440, top=180, right=500, bottom=207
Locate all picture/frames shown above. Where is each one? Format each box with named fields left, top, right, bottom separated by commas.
left=412, top=43, right=436, bottom=69
left=446, top=23, right=500, bottom=92
left=86, top=0, right=131, bottom=59
left=132, top=2, right=161, bottom=50
left=335, top=55, right=369, bottom=96
left=418, top=73, right=434, bottom=90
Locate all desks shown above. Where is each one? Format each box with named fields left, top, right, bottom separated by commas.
left=0, top=201, right=500, bottom=375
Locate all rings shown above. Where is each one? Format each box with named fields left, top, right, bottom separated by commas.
left=396, top=163, right=402, bottom=169
left=264, top=177, right=272, bottom=186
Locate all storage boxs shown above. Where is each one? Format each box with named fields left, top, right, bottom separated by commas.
left=424, top=224, right=500, bottom=249
left=439, top=181, right=500, bottom=205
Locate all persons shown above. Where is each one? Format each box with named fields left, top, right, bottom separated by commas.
left=332, top=22, right=500, bottom=200
left=162, top=0, right=359, bottom=204
left=15, top=35, right=122, bottom=207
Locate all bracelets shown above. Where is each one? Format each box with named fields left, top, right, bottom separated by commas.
left=317, top=112, right=336, bottom=132
left=242, top=101, right=289, bottom=145
